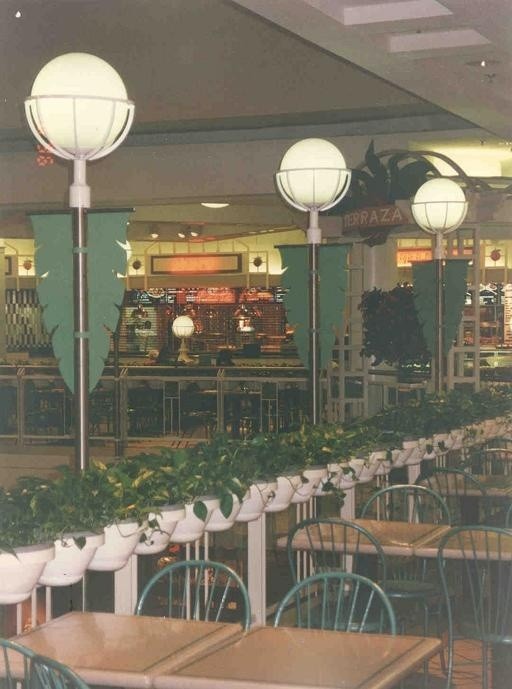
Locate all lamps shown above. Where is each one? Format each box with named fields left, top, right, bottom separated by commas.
left=145, top=221, right=161, bottom=242
left=171, top=315, right=195, bottom=362
left=176, top=223, right=204, bottom=242
